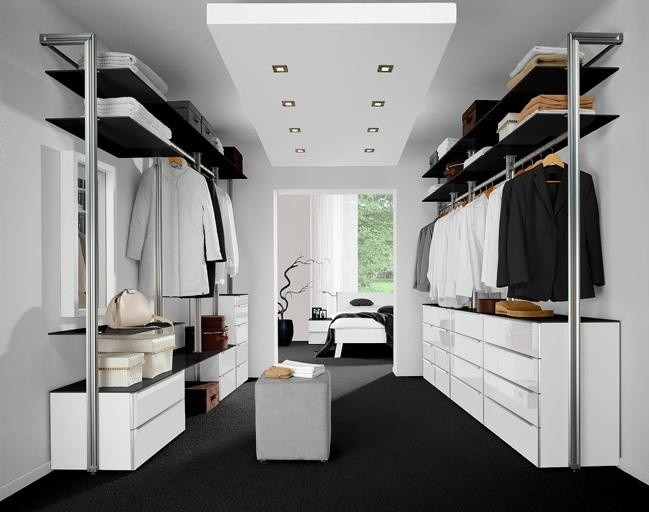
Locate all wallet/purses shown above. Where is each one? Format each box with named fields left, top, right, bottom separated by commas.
left=265, top=366, right=292, bottom=379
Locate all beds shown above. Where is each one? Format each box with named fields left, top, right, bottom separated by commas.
left=334, top=290, right=393, bottom=359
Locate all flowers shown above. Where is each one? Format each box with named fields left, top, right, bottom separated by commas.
left=516, top=93, right=595, bottom=122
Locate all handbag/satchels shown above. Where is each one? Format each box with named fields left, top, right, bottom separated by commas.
left=105, top=289, right=152, bottom=329
left=495, top=301, right=553, bottom=318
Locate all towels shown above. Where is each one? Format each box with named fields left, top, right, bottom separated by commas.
left=91, top=93, right=174, bottom=140
left=506, top=45, right=585, bottom=88
left=75, top=49, right=169, bottom=100
left=275, top=359, right=327, bottom=379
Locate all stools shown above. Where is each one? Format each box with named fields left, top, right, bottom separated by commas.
left=252, top=364, right=333, bottom=464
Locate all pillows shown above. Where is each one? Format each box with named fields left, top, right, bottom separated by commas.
left=345, top=305, right=379, bottom=312
left=348, top=298, right=374, bottom=306
left=377, top=305, right=393, bottom=314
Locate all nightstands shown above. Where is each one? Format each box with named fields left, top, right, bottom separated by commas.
left=308, top=318, right=332, bottom=345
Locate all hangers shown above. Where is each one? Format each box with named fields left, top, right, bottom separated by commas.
left=165, top=156, right=183, bottom=169
left=188, top=159, right=212, bottom=179
left=436, top=142, right=566, bottom=217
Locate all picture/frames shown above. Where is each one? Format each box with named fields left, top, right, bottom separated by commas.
left=312, top=307, right=323, bottom=319
left=60, top=149, right=117, bottom=318
left=319, top=310, right=327, bottom=320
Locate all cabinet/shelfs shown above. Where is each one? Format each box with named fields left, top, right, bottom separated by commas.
left=47, top=317, right=185, bottom=472
left=185, top=292, right=250, bottom=403
left=39, top=30, right=248, bottom=181
left=421, top=302, right=623, bottom=467
left=422, top=31, right=624, bottom=203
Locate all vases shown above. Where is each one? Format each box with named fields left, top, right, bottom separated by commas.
left=278, top=318, right=295, bottom=346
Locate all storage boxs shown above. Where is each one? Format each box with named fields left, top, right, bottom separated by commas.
left=476, top=298, right=506, bottom=313
left=496, top=112, right=520, bottom=142
left=200, top=332, right=229, bottom=350
left=224, top=145, right=243, bottom=173
left=97, top=351, right=145, bottom=388
left=461, top=99, right=501, bottom=135
left=87, top=333, right=176, bottom=380
left=169, top=100, right=202, bottom=134
left=428, top=151, right=439, bottom=169
left=184, top=382, right=220, bottom=414
left=201, top=115, right=213, bottom=142
left=200, top=313, right=227, bottom=334
